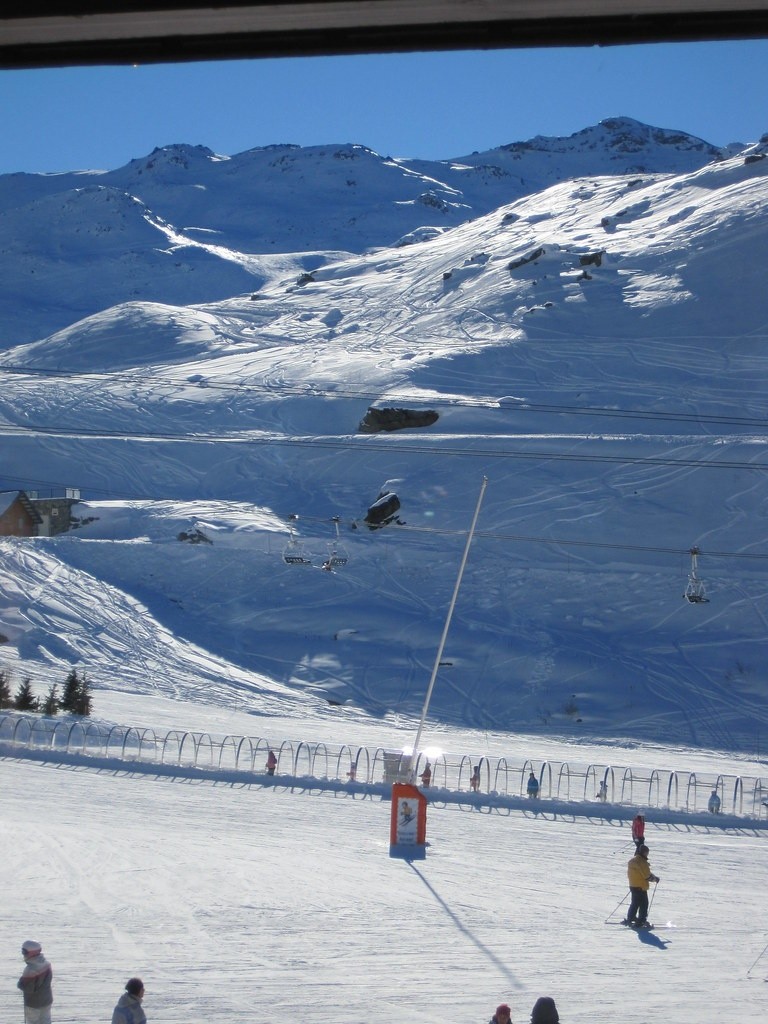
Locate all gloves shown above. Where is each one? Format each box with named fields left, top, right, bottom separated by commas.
left=635, top=839, right=639, bottom=842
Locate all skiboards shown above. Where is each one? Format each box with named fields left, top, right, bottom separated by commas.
left=605, top=920, right=668, bottom=931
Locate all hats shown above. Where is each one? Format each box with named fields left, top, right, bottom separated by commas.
left=497, top=1005, right=511, bottom=1017
left=125, top=978, right=143, bottom=996
left=636, top=809, right=646, bottom=817
left=21, top=940, right=41, bottom=952
left=639, top=844, right=650, bottom=855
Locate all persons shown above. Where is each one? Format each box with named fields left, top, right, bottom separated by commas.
left=112, top=978, right=148, bottom=1024
left=530, top=996, right=561, bottom=1024
left=470, top=766, right=480, bottom=792
left=526, top=773, right=539, bottom=797
left=708, top=791, right=720, bottom=812
left=17, top=941, right=54, bottom=1024
left=266, top=750, right=277, bottom=775
left=418, top=762, right=432, bottom=787
left=346, top=762, right=357, bottom=781
left=488, top=1003, right=513, bottom=1024
left=596, top=781, right=607, bottom=802
left=625, top=845, right=659, bottom=929
left=632, top=808, right=645, bottom=853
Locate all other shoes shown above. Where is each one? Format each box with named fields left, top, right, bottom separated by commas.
left=627, top=916, right=646, bottom=923
